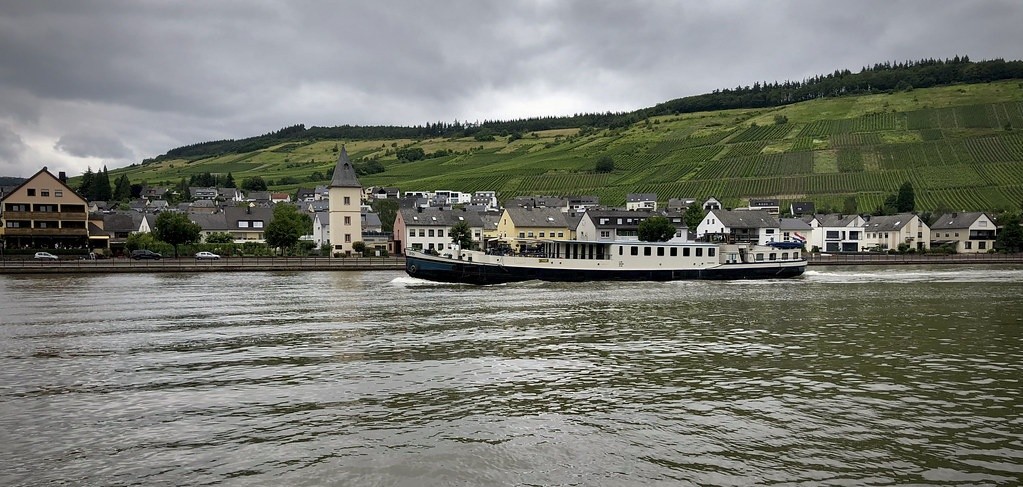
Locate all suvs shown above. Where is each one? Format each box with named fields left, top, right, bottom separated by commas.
left=132, top=250, right=162, bottom=260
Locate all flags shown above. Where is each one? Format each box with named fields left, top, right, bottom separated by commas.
left=793, top=231, right=806, bottom=242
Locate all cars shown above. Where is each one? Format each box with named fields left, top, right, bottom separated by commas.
left=34, top=252, right=58, bottom=261
left=194, top=251, right=220, bottom=260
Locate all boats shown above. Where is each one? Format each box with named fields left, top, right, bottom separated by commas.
left=403, top=225, right=809, bottom=286
